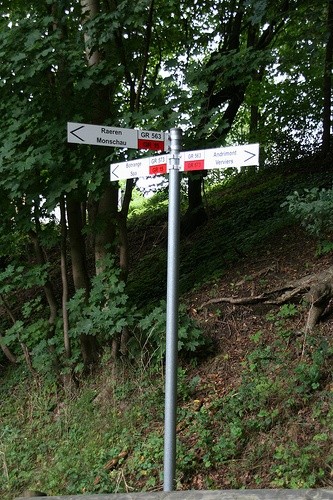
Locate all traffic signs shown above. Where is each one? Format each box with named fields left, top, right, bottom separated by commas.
left=67, top=121, right=172, bottom=153
left=110, top=154, right=170, bottom=182
left=178, top=143, right=260, bottom=172
